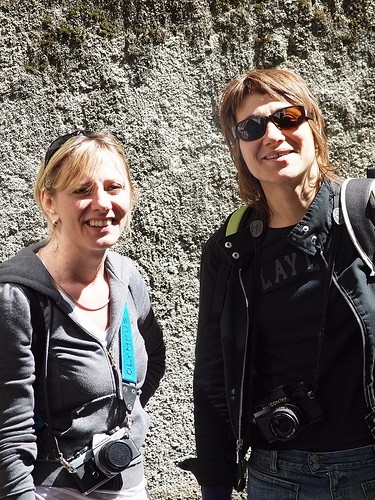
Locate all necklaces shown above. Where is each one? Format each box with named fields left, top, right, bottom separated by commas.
left=41, top=260, right=111, bottom=311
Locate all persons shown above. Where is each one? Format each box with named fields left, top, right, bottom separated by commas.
left=180, top=69, right=375, bottom=500
left=0, top=130, right=166, bottom=499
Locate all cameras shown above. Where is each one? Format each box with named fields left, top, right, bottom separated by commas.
left=67, top=426, right=141, bottom=496
left=252, top=382, right=323, bottom=443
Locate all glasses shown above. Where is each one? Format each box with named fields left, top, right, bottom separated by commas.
left=233, top=105, right=313, bottom=141
left=44, top=130, right=97, bottom=167
